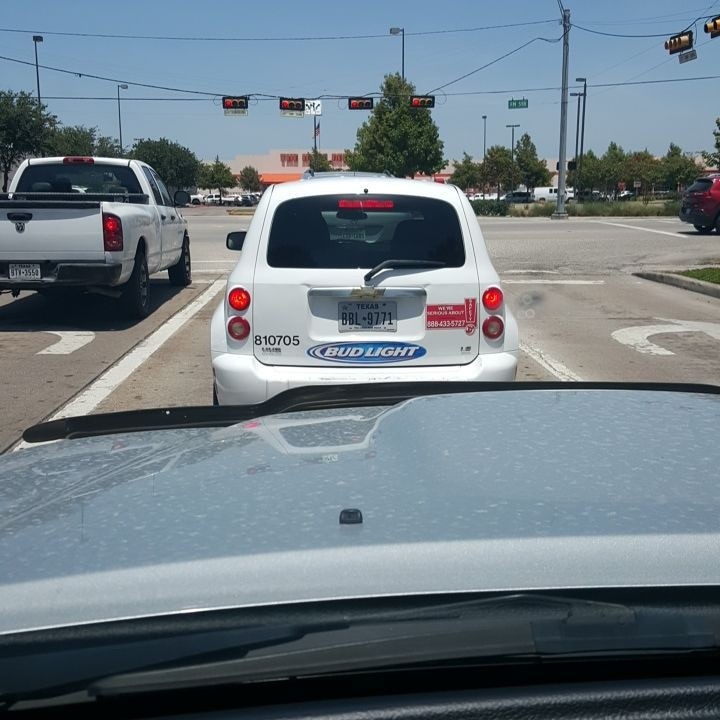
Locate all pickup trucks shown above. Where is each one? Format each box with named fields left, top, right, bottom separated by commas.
left=0, top=151, right=193, bottom=321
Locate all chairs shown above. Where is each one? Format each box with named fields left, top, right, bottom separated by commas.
left=279, top=210, right=330, bottom=240
left=390, top=219, right=438, bottom=247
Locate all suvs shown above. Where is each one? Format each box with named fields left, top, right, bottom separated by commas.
left=678, top=172, right=720, bottom=237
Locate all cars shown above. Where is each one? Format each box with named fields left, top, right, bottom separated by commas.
left=567, top=187, right=636, bottom=201
left=300, top=168, right=414, bottom=248
left=503, top=191, right=533, bottom=203
left=188, top=191, right=262, bottom=207
left=208, top=174, right=520, bottom=414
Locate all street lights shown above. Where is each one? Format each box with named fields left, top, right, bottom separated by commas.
left=32, top=35, right=44, bottom=117
left=390, top=28, right=405, bottom=88
left=482, top=115, right=487, bottom=200
left=575, top=77, right=587, bottom=200
left=506, top=124, right=521, bottom=202
left=117, top=84, right=128, bottom=158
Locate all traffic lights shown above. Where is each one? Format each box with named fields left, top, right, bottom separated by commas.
left=279, top=97, right=305, bottom=111
left=409, top=95, right=435, bottom=108
left=349, top=98, right=374, bottom=109
left=664, top=30, right=693, bottom=54
left=703, top=15, right=720, bottom=39
left=222, top=96, right=248, bottom=109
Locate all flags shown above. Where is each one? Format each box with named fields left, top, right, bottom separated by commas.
left=312, top=121, right=320, bottom=138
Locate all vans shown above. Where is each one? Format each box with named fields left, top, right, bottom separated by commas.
left=533, top=186, right=569, bottom=203
left=569, top=91, right=587, bottom=199
